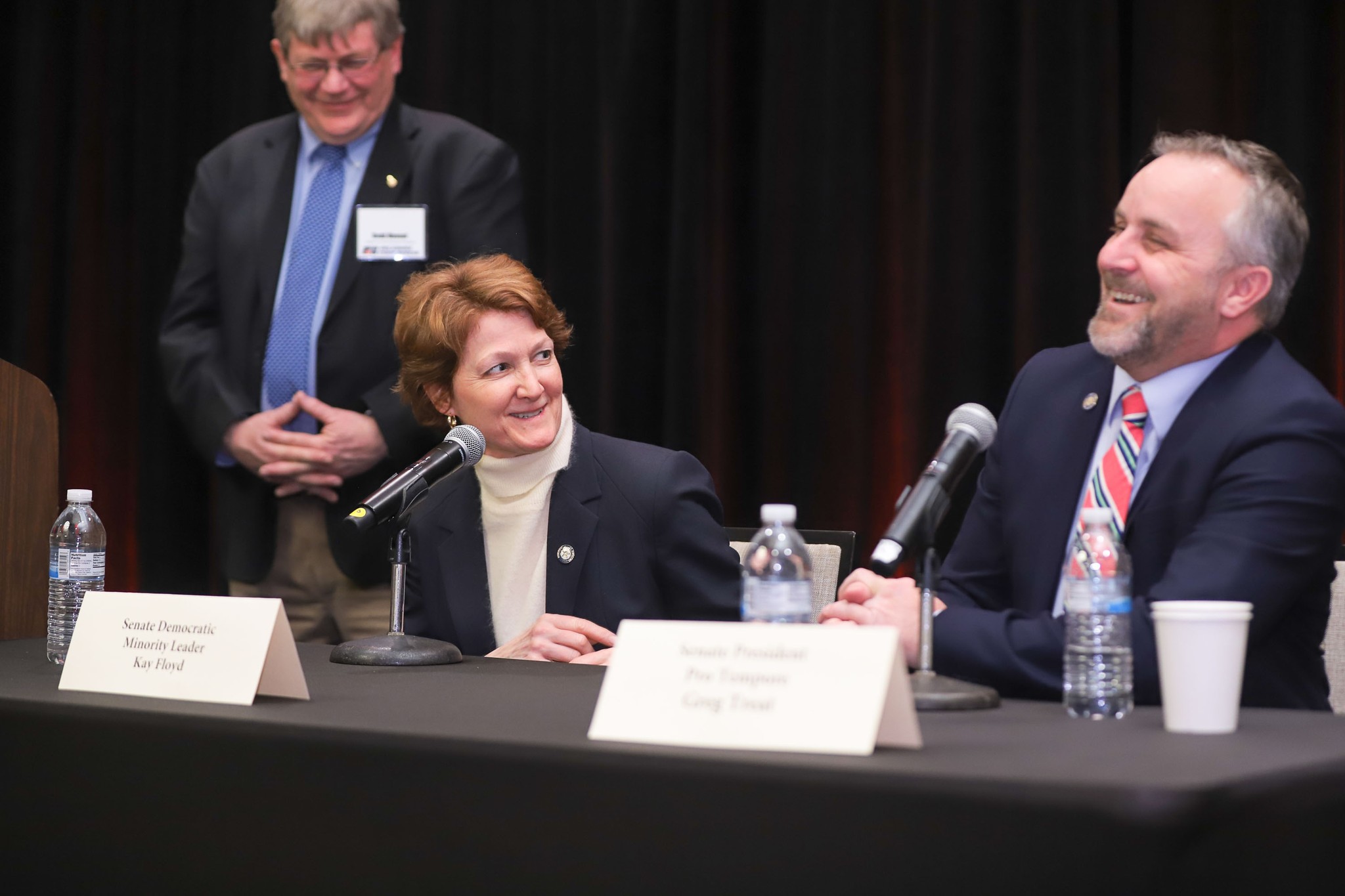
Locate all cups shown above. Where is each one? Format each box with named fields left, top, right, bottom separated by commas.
left=1149, top=601, right=1255, bottom=735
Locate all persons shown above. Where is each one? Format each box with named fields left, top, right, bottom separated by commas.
left=817, top=128, right=1345, bottom=711
left=391, top=251, right=742, bottom=666
left=159, top=0, right=527, bottom=643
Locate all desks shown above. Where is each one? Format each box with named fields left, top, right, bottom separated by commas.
left=0, top=636, right=1345, bottom=896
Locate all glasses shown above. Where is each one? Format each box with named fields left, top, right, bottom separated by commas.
left=285, top=45, right=387, bottom=79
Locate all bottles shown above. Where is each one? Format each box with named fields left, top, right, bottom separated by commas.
left=1063, top=508, right=1136, bottom=721
left=47, top=490, right=108, bottom=665
left=741, top=504, right=813, bottom=624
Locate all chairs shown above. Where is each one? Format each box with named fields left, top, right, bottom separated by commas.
left=1, top=359, right=59, bottom=634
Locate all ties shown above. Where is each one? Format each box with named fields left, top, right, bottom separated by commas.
left=265, top=143, right=348, bottom=436
left=1066, top=389, right=1148, bottom=587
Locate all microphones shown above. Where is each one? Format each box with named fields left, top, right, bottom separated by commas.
left=869, top=401, right=1000, bottom=575
left=342, top=424, right=485, bottom=535
